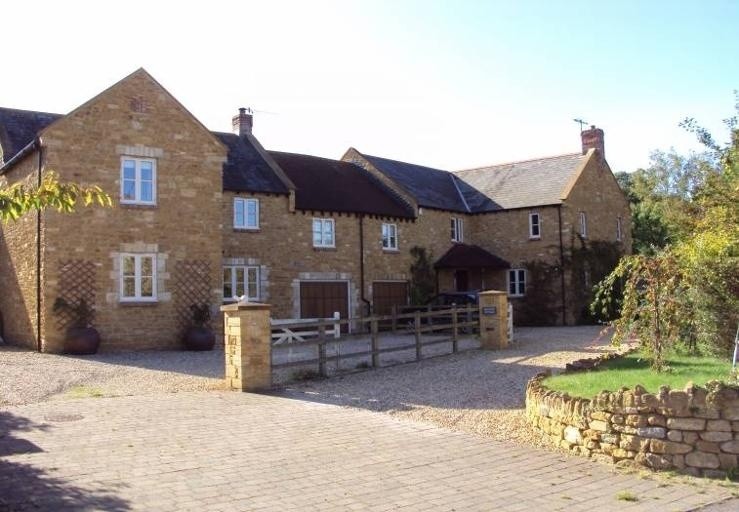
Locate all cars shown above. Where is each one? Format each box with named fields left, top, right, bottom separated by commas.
left=400, top=292, right=481, bottom=336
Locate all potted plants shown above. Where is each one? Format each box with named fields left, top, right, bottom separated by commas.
left=183, top=301, right=214, bottom=351
left=53, top=297, right=99, bottom=353
